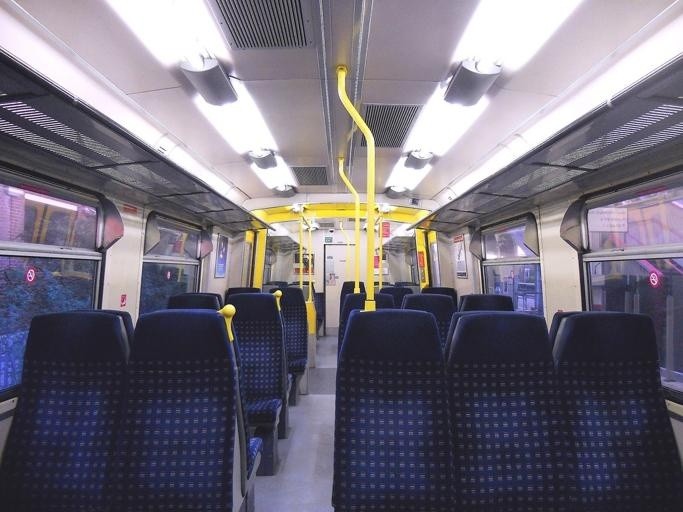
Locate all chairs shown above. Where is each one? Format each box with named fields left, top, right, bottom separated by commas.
left=446, top=313, right=553, bottom=512
left=0, top=312, right=130, bottom=512
left=114, top=308, right=238, bottom=511
left=164, top=281, right=515, bottom=511
left=550, top=312, right=683, bottom=511
